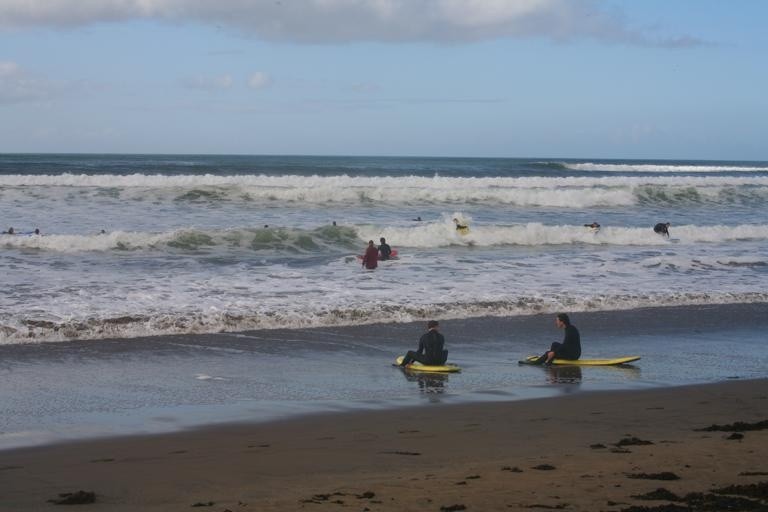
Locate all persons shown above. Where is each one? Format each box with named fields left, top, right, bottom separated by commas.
left=361, top=240, right=379, bottom=269
left=376, top=237, right=391, bottom=261
left=452, top=216, right=467, bottom=230
left=517, top=312, right=582, bottom=366
left=534, top=364, right=582, bottom=392
left=398, top=367, right=448, bottom=402
left=584, top=221, right=600, bottom=228
left=654, top=220, right=671, bottom=240
left=391, top=320, right=448, bottom=368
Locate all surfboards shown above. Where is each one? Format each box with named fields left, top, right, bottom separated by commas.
left=526, top=356, right=641, bottom=365
left=396, top=356, right=461, bottom=372
